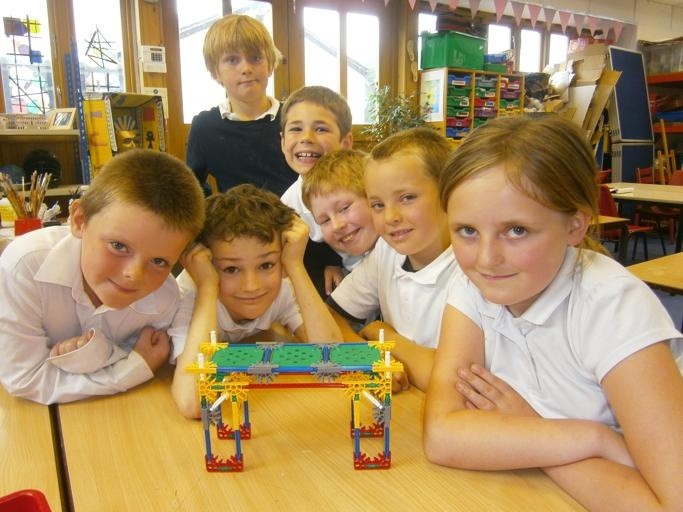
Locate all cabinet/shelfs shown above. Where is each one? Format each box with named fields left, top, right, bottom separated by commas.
left=1, top=129, right=84, bottom=201
left=646, top=74, right=682, bottom=184
left=415, top=70, right=523, bottom=138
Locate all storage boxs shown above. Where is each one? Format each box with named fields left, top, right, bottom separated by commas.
left=500, top=98, right=520, bottom=109
left=475, top=75, right=495, bottom=88
left=475, top=108, right=496, bottom=118
left=499, top=109, right=520, bottom=117
left=445, top=96, right=470, bottom=108
left=473, top=97, right=496, bottom=106
left=641, top=42, right=682, bottom=74
left=446, top=116, right=470, bottom=128
left=447, top=86, right=471, bottom=97
left=501, top=79, right=521, bottom=91
left=447, top=75, right=470, bottom=87
left=500, top=89, right=520, bottom=99
left=418, top=31, right=485, bottom=71
left=474, top=118, right=494, bottom=128
left=445, top=126, right=470, bottom=138
left=447, top=106, right=470, bottom=118
left=475, top=86, right=495, bottom=98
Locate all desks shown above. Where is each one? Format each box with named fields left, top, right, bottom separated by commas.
left=624, top=248, right=683, bottom=331
left=600, top=182, right=683, bottom=249
left=586, top=215, right=628, bottom=265
left=0, top=360, right=585, bottom=512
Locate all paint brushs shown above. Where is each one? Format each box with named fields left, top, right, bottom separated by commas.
left=1, top=170, right=53, bottom=218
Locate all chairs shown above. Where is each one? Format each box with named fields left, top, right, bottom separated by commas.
left=634, top=166, right=675, bottom=257
left=598, top=184, right=652, bottom=263
left=595, top=169, right=612, bottom=185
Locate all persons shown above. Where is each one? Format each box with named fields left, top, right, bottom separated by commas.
left=0, top=148, right=207, bottom=406
left=301, top=149, right=382, bottom=303
left=164, top=183, right=345, bottom=421
left=420, top=111, right=683, bottom=511
left=184, top=13, right=299, bottom=201
left=279, top=85, right=353, bottom=244
left=325, top=125, right=456, bottom=393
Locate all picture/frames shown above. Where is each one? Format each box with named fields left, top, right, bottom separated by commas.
left=47, top=108, right=75, bottom=129
